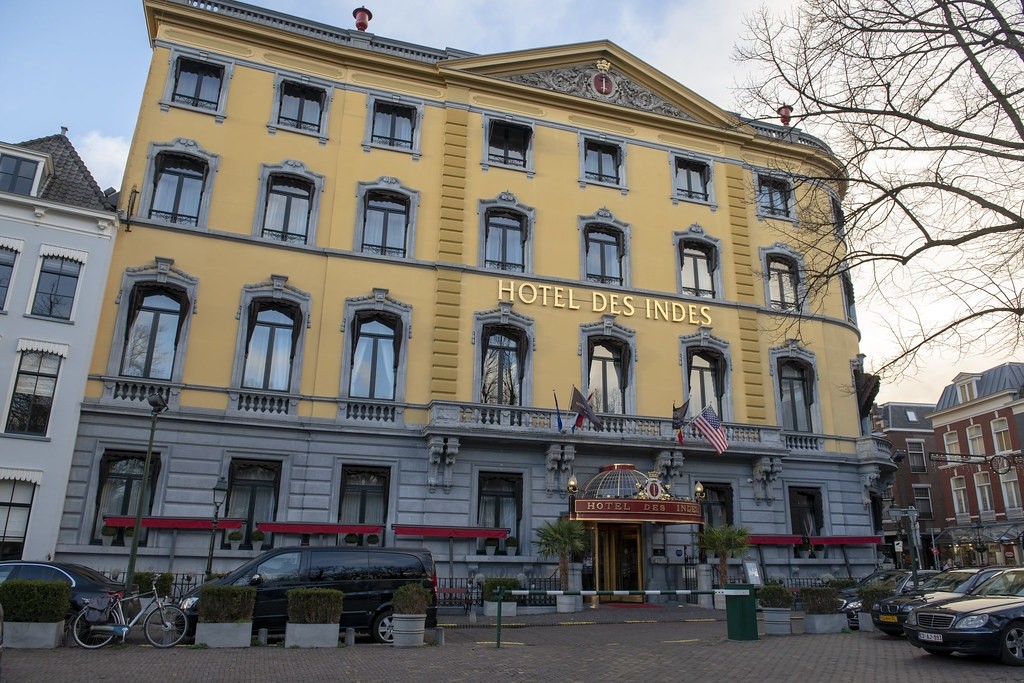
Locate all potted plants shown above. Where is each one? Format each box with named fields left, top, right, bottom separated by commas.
left=192, top=585, right=260, bottom=649
left=527, top=516, right=582, bottom=614
left=282, top=585, right=346, bottom=650
left=482, top=577, right=517, bottom=616
left=127, top=572, right=174, bottom=615
left=695, top=520, right=753, bottom=611
left=857, top=584, right=895, bottom=632
left=391, top=583, right=429, bottom=648
left=3, top=576, right=71, bottom=650
left=759, top=585, right=795, bottom=636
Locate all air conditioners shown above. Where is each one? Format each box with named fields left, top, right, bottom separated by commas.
left=799, top=586, right=850, bottom=633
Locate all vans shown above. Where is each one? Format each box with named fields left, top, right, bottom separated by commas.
left=176, top=545, right=438, bottom=643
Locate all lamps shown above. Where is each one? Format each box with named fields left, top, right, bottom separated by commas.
left=567, top=469, right=578, bottom=496
left=693, top=479, right=705, bottom=502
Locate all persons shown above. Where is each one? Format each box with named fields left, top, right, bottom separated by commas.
left=943, top=559, right=958, bottom=571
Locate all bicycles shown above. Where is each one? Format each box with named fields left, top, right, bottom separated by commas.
left=73, top=570, right=190, bottom=649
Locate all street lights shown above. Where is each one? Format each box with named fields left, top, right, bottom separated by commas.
left=886, top=496, right=906, bottom=569
left=203, top=475, right=229, bottom=583
left=972, top=520, right=985, bottom=566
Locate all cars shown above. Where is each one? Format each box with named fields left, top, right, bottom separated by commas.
left=869, top=566, right=991, bottom=639
left=832, top=569, right=942, bottom=629
left=904, top=567, right=1024, bottom=666
left=0, top=560, right=142, bottom=629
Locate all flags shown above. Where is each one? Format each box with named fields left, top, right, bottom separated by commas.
left=693, top=404, right=729, bottom=454
left=554, top=387, right=603, bottom=433
left=672, top=398, right=689, bottom=444
